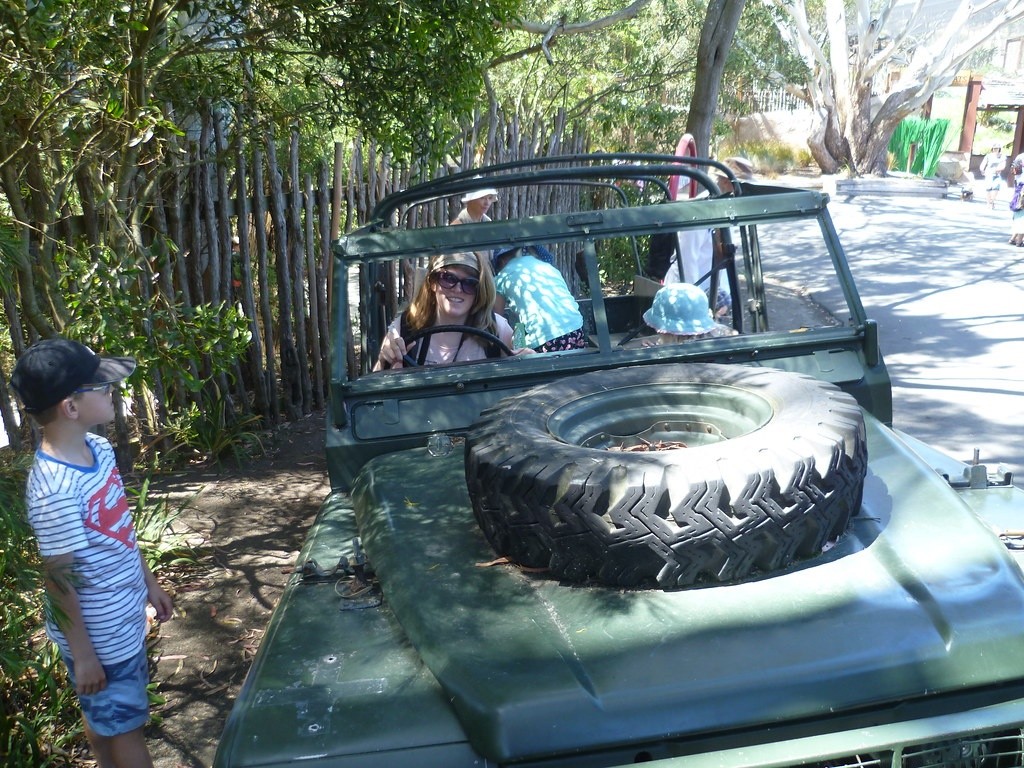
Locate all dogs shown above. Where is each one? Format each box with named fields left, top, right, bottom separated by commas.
left=961, top=187, right=974, bottom=202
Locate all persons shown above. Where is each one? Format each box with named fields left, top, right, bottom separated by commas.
left=641, top=282, right=719, bottom=346
left=373, top=252, right=537, bottom=372
left=492, top=248, right=585, bottom=354
left=1008, top=152, right=1024, bottom=247
left=662, top=157, right=761, bottom=295
left=979, top=142, right=1006, bottom=210
left=10, top=338, right=173, bottom=768
left=451, top=188, right=499, bottom=227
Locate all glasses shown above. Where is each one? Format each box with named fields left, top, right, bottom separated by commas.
left=432, top=272, right=480, bottom=295
left=74, top=384, right=110, bottom=396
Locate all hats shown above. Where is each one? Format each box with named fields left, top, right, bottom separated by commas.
left=430, top=252, right=480, bottom=275
left=490, top=243, right=554, bottom=264
left=10, top=339, right=137, bottom=414
left=642, top=283, right=717, bottom=336
left=461, top=177, right=498, bottom=203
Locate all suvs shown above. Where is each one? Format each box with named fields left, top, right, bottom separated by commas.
left=213, top=152, right=1023, bottom=766
